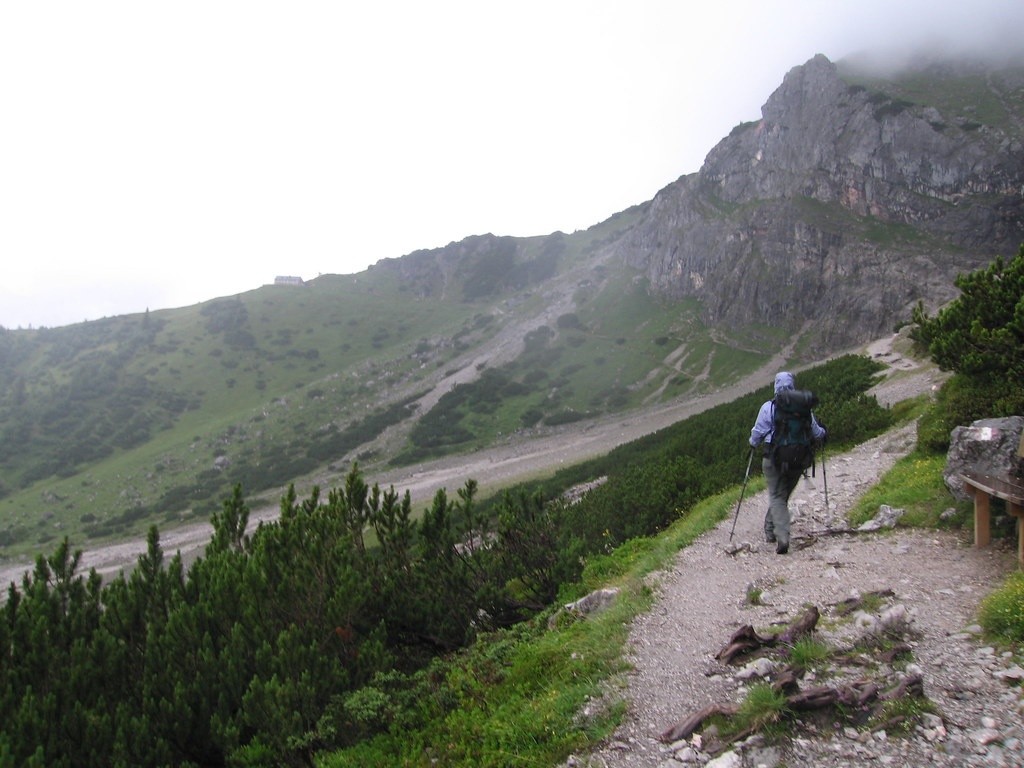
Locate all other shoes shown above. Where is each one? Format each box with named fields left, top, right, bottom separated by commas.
left=766, top=532, right=776, bottom=543
left=776, top=540, right=789, bottom=554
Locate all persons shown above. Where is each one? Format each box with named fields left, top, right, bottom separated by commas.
left=748, top=372, right=828, bottom=554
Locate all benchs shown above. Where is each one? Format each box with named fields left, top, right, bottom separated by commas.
left=958, top=429, right=1024, bottom=564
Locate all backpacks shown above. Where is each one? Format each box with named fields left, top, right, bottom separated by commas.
left=768, top=390, right=816, bottom=479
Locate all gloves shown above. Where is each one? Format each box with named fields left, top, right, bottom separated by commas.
left=817, top=421, right=827, bottom=433
